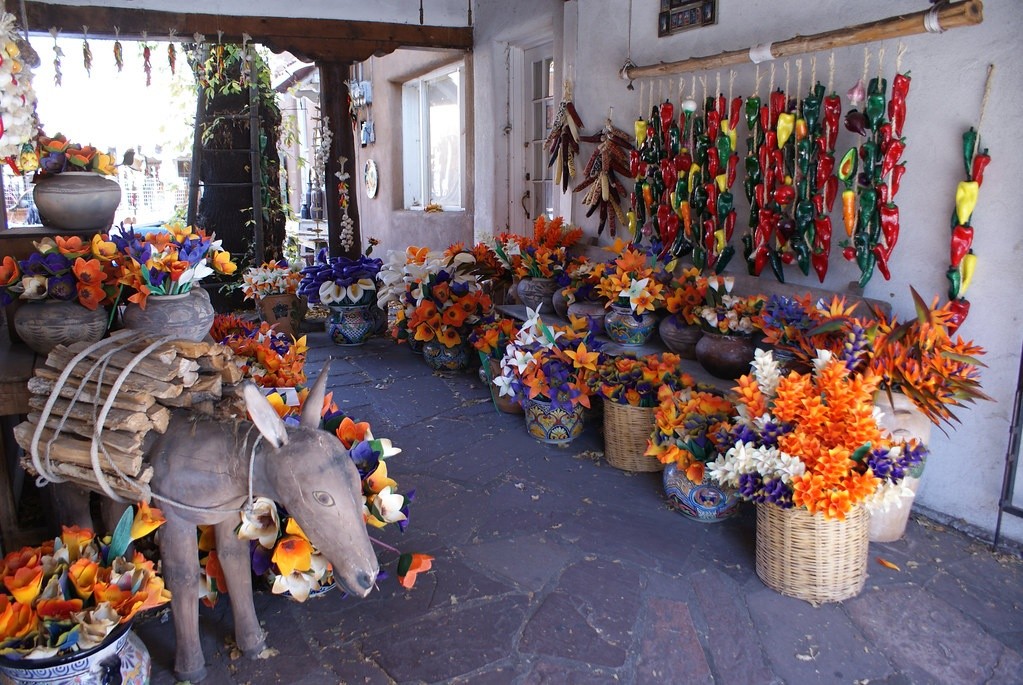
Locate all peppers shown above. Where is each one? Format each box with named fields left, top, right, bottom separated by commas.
left=338, top=181, right=349, bottom=207
left=626, top=70, right=911, bottom=287
left=946, top=126, right=992, bottom=336
left=53, top=40, right=223, bottom=88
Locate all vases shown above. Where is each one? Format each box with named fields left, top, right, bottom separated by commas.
left=660, top=310, right=697, bottom=359
left=33, top=173, right=122, bottom=234
left=568, top=300, right=602, bottom=331
left=604, top=300, right=657, bottom=345
left=323, top=304, right=376, bottom=347
left=0, top=621, right=152, bottom=685
left=521, top=387, right=585, bottom=444
left=755, top=498, right=871, bottom=603
left=13, top=296, right=107, bottom=353
left=260, top=291, right=304, bottom=344
left=515, top=276, right=553, bottom=315
left=401, top=327, right=421, bottom=356
left=125, top=292, right=217, bottom=341
left=662, top=461, right=739, bottom=523
left=873, top=480, right=914, bottom=544
left=605, top=396, right=664, bottom=472
left=487, top=358, right=524, bottom=413
left=755, top=345, right=795, bottom=386
left=697, top=330, right=753, bottom=382
left=423, top=329, right=467, bottom=374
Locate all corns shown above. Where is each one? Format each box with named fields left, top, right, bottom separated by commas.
left=543, top=101, right=636, bottom=239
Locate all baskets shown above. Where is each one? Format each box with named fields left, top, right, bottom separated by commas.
left=603, top=397, right=666, bottom=475
left=757, top=487, right=869, bottom=605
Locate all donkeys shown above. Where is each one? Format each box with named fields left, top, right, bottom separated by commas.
left=56, top=351, right=380, bottom=678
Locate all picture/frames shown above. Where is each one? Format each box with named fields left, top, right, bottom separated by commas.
left=555, top=285, right=579, bottom=316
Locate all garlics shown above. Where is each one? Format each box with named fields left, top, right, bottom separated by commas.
left=321, top=126, right=332, bottom=162
left=339, top=213, right=354, bottom=252
left=316, top=153, right=326, bottom=186
left=0, top=59, right=32, bottom=149
left=335, top=171, right=350, bottom=181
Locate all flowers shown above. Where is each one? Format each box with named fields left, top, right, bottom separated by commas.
left=1, top=133, right=1001, bottom=660
